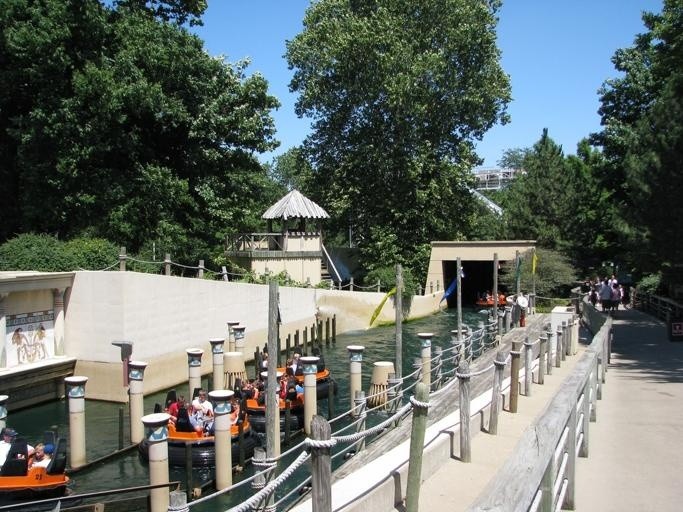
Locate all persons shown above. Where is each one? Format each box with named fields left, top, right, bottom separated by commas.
left=480, top=289, right=507, bottom=301
left=0, top=426, right=32, bottom=469
left=27, top=442, right=44, bottom=467
left=157, top=341, right=326, bottom=438
left=31, top=443, right=55, bottom=467
left=581, top=273, right=626, bottom=312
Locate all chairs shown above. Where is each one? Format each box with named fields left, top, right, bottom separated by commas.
left=154, top=347, right=325, bottom=433
left=0, top=430, right=67, bottom=476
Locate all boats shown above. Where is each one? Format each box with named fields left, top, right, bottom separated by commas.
left=139, top=356, right=337, bottom=466
left=0, top=431, right=69, bottom=494
left=478, top=294, right=507, bottom=305
